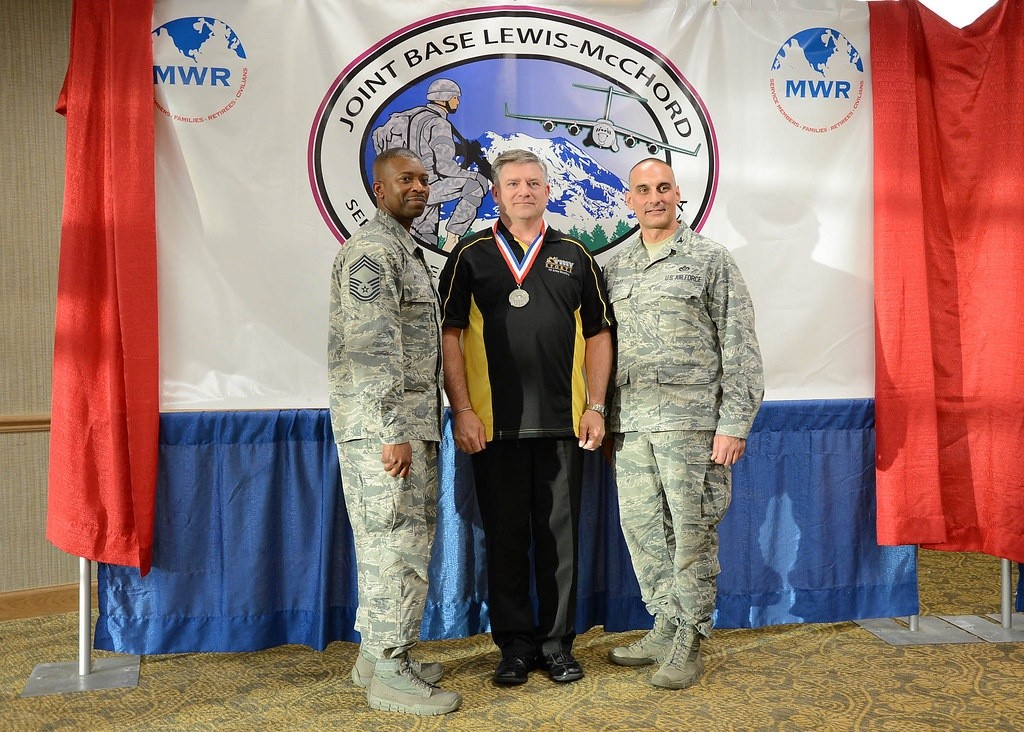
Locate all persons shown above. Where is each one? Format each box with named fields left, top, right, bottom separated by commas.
left=438, top=146, right=618, bottom=690
left=326, top=147, right=463, bottom=716
left=601, top=159, right=766, bottom=688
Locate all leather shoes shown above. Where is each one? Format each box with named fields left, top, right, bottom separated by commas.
left=494, top=649, right=584, bottom=685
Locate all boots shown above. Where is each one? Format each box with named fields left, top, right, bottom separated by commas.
left=609, top=612, right=704, bottom=689
left=353, top=645, right=462, bottom=716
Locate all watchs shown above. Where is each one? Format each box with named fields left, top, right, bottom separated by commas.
left=585, top=404, right=608, bottom=419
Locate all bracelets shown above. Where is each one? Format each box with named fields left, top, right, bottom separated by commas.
left=453, top=408, right=473, bottom=415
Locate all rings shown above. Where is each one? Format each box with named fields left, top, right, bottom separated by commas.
left=598, top=443, right=602, bottom=447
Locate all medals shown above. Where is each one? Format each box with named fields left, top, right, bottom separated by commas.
left=508, top=288, right=530, bottom=309
